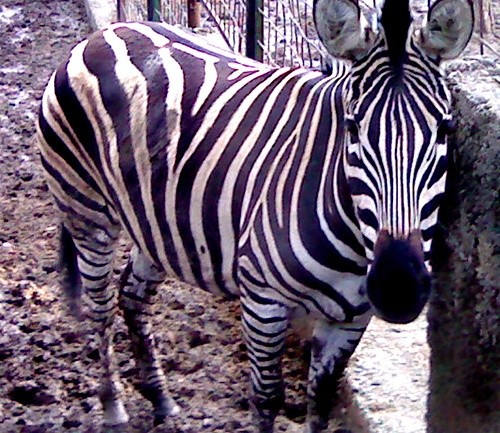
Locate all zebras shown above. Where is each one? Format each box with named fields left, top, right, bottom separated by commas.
left=35, top=0, right=475, bottom=433
